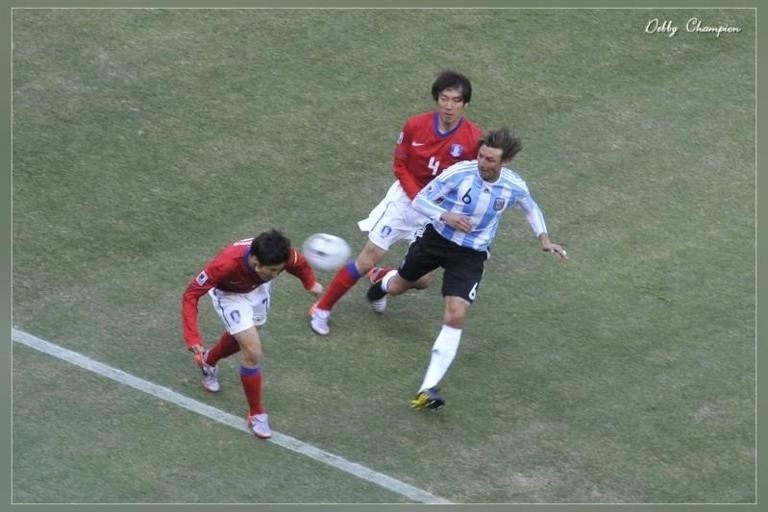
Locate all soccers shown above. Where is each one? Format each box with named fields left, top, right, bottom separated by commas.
left=304, top=232, right=350, bottom=270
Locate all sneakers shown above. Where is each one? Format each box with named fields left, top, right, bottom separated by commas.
left=194, top=350, right=219, bottom=392
left=309, top=303, right=331, bottom=335
left=367, top=266, right=399, bottom=314
left=409, top=388, right=445, bottom=411
left=247, top=410, right=271, bottom=438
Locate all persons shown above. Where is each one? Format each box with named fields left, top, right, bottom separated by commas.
left=308, top=70, right=484, bottom=334
left=366, top=127, right=568, bottom=411
left=178, top=227, right=324, bottom=439
